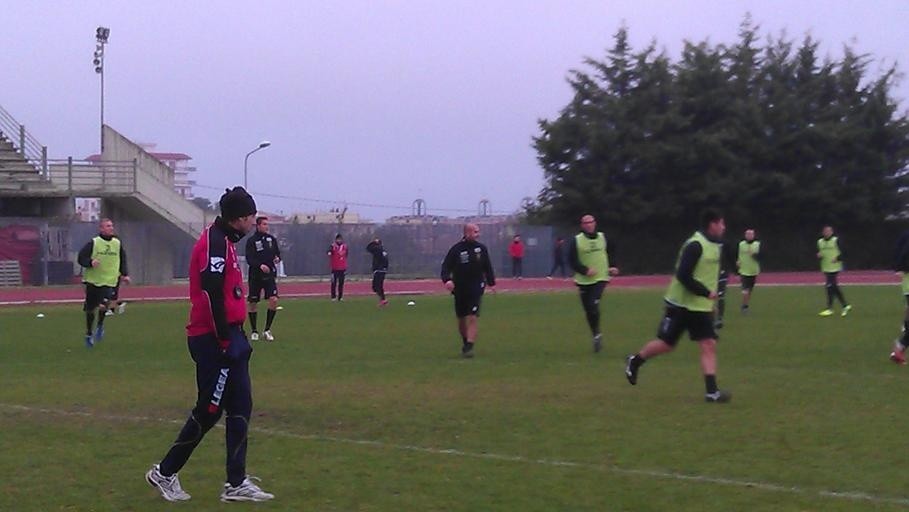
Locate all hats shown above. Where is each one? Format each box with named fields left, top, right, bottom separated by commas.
left=220, top=187, right=256, bottom=222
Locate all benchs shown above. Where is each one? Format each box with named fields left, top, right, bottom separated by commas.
left=0, top=131, right=50, bottom=187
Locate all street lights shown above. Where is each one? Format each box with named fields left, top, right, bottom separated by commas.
left=431, top=215, right=439, bottom=257
left=244, top=140, right=271, bottom=187
left=91, top=25, right=110, bottom=154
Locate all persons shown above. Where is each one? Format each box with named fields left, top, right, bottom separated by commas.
left=568, top=213, right=621, bottom=352
left=244, top=216, right=281, bottom=342
left=814, top=224, right=853, bottom=318
left=327, top=233, right=349, bottom=302
left=508, top=235, right=527, bottom=279
left=546, top=239, right=570, bottom=279
left=890, top=229, right=908, bottom=365
left=440, top=223, right=499, bottom=356
left=366, top=238, right=390, bottom=307
left=734, top=229, right=765, bottom=313
left=624, top=210, right=735, bottom=403
left=714, top=233, right=730, bottom=327
left=144, top=184, right=277, bottom=504
left=78, top=218, right=130, bottom=348
left=105, top=273, right=127, bottom=315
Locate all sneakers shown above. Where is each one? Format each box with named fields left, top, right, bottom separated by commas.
left=705, top=389, right=731, bottom=402
left=144, top=464, right=191, bottom=502
left=264, top=330, right=274, bottom=341
left=625, top=352, right=644, bottom=385
left=105, top=310, right=114, bottom=316
left=251, top=333, right=259, bottom=341
left=819, top=310, right=833, bottom=317
left=841, top=304, right=852, bottom=317
left=220, top=477, right=274, bottom=503
left=119, top=302, right=126, bottom=314
